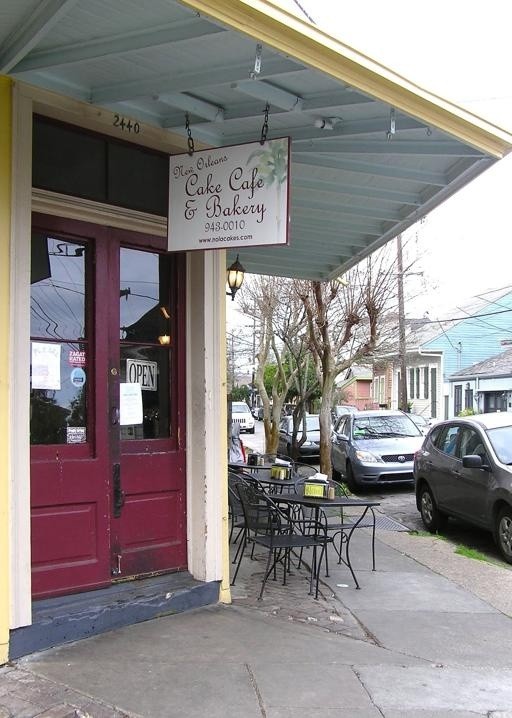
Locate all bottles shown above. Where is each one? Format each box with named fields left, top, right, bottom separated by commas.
left=259, top=458, right=264, bottom=466
left=328, top=487, right=334, bottom=500
left=279, top=469, right=284, bottom=480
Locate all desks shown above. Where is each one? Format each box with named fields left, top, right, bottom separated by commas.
left=256, top=493, right=380, bottom=579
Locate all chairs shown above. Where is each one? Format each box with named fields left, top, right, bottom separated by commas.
left=227, top=452, right=375, bottom=605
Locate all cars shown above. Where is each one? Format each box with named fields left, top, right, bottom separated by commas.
left=232, top=401, right=309, bottom=434
left=276, top=414, right=337, bottom=466
left=329, top=409, right=426, bottom=496
left=406, top=412, right=433, bottom=438
left=330, top=406, right=359, bottom=428
left=412, top=411, right=512, bottom=566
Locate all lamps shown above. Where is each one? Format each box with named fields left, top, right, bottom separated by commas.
left=223, top=249, right=247, bottom=300
